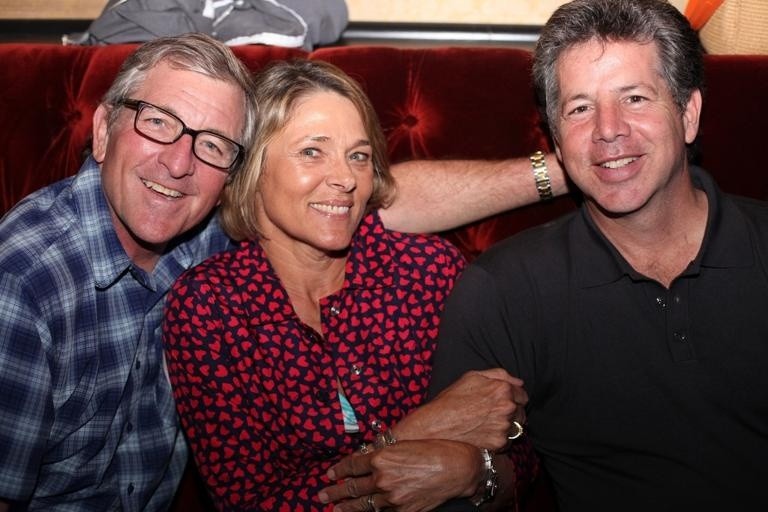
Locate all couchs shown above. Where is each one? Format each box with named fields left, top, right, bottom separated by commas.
left=0, top=34, right=768, bottom=275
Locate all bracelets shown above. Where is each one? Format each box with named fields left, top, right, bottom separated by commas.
left=371, top=424, right=397, bottom=446
left=528, top=148, right=561, bottom=200
left=468, top=443, right=505, bottom=507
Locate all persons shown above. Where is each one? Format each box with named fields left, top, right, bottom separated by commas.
left=161, top=60, right=535, bottom=512
left=4, top=32, right=576, bottom=511
left=424, top=0, right=767, bottom=512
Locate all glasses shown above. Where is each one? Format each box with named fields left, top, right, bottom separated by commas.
left=113, top=98, right=247, bottom=171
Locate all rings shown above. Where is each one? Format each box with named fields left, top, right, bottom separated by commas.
left=507, top=419, right=525, bottom=440
left=367, top=493, right=383, bottom=511
